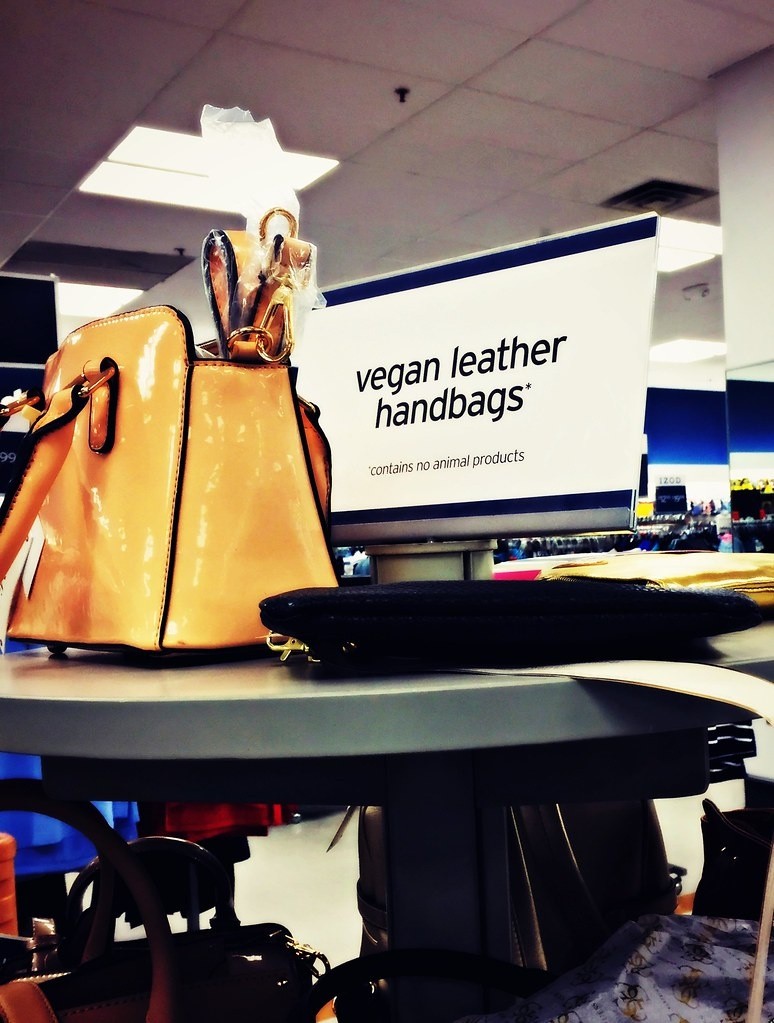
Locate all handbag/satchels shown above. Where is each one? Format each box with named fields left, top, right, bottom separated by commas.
left=0, top=208, right=343, bottom=660
left=260, top=582, right=762, bottom=661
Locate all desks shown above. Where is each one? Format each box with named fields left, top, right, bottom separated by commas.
left=0, top=625, right=774, bottom=1023
